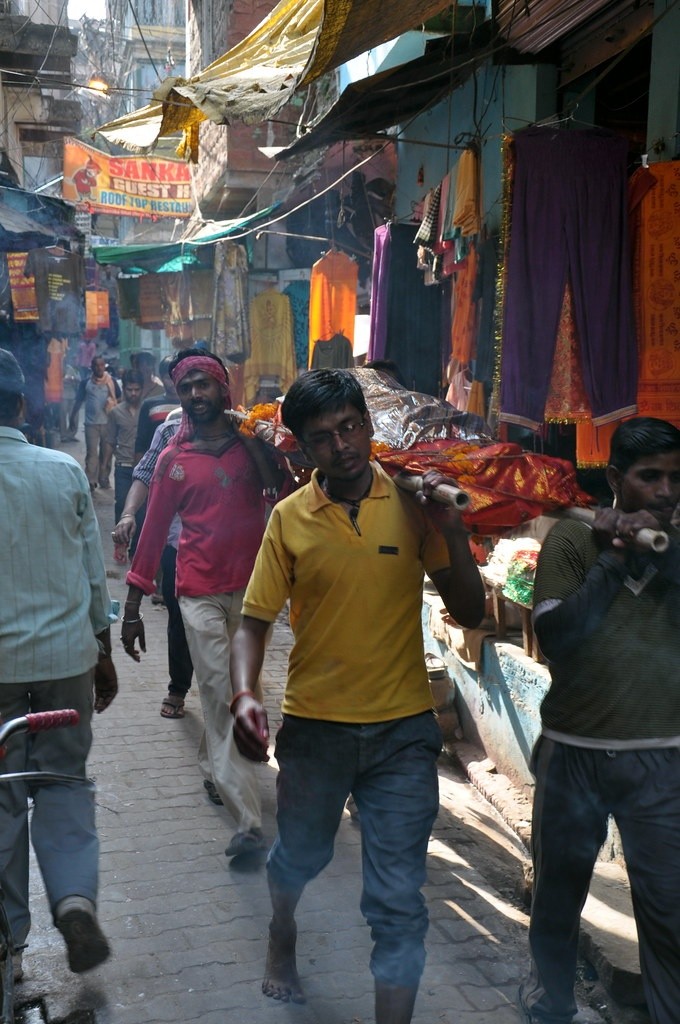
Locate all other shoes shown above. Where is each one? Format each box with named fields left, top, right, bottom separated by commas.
left=204, top=779, right=223, bottom=805
left=224, top=829, right=267, bottom=855
left=55, top=896, right=109, bottom=973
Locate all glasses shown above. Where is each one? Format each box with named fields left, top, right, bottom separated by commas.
left=301, top=414, right=366, bottom=450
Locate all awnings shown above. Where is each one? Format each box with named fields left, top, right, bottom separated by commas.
left=87, top=0, right=492, bottom=273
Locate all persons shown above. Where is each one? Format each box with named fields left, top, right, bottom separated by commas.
left=60, top=331, right=245, bottom=719
left=518, top=416, right=680, bottom=1024
left=0, top=348, right=118, bottom=984
left=229, top=367, right=486, bottom=1024
left=120, top=348, right=296, bottom=805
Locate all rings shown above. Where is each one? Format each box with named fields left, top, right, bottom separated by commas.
left=120, top=636, right=123, bottom=639
left=124, top=645, right=128, bottom=648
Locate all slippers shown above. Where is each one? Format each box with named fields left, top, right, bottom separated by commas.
left=161, top=698, right=185, bottom=718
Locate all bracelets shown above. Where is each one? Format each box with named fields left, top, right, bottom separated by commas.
left=226, top=690, right=257, bottom=715
left=121, top=612, right=144, bottom=623
